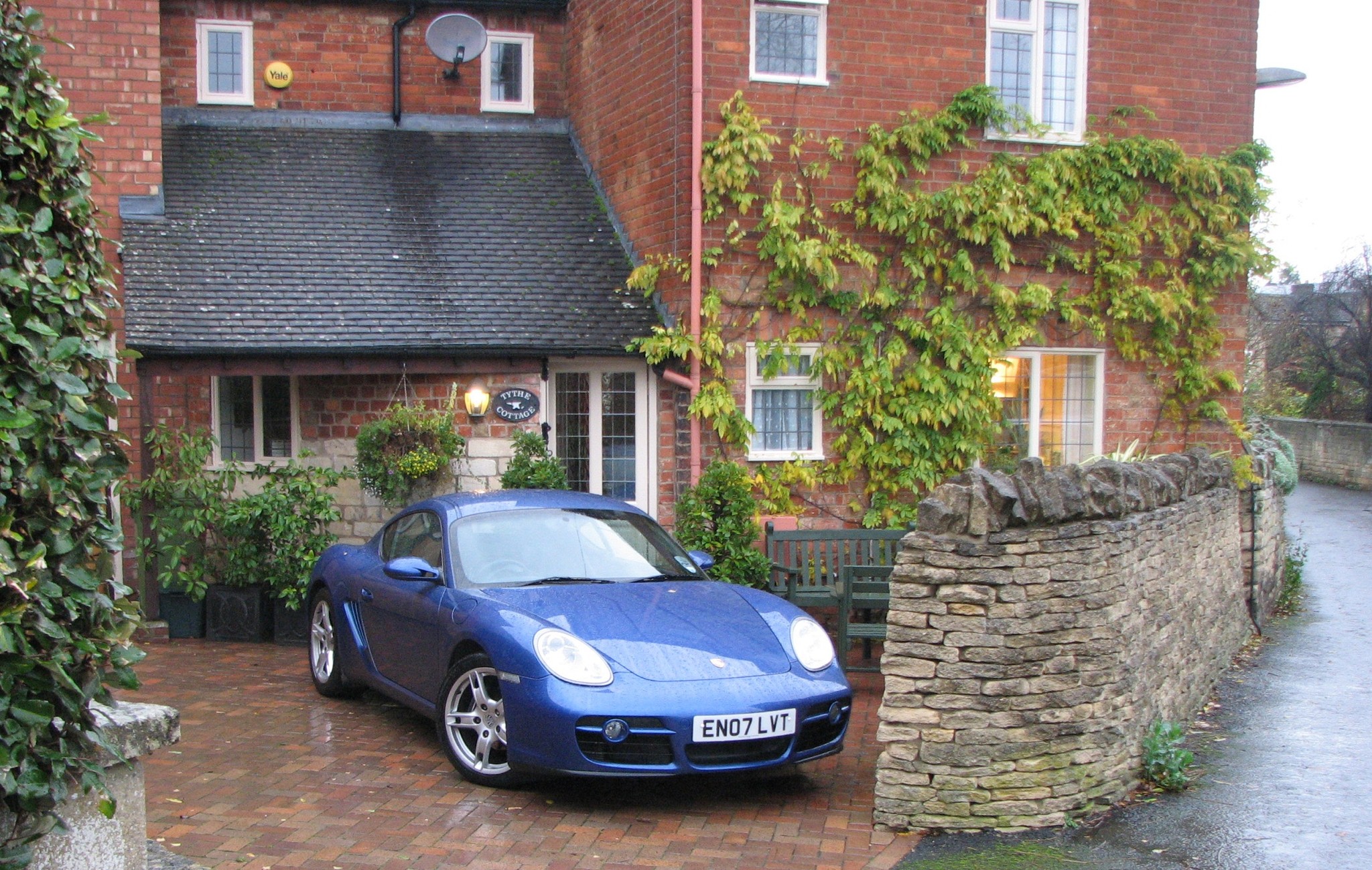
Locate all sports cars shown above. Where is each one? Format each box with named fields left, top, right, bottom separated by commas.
left=306, top=488, right=855, bottom=788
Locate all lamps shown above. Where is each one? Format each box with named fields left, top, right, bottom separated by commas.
left=463, top=374, right=491, bottom=417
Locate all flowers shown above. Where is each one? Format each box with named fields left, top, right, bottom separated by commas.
left=348, top=423, right=440, bottom=514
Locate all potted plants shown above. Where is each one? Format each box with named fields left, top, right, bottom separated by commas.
left=115, top=419, right=226, bottom=638
left=252, top=453, right=356, bottom=647
left=201, top=496, right=273, bottom=643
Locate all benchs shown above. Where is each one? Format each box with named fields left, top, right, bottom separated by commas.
left=762, top=521, right=916, bottom=659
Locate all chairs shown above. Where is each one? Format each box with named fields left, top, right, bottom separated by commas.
left=829, top=565, right=896, bottom=677
left=402, top=533, right=442, bottom=581
